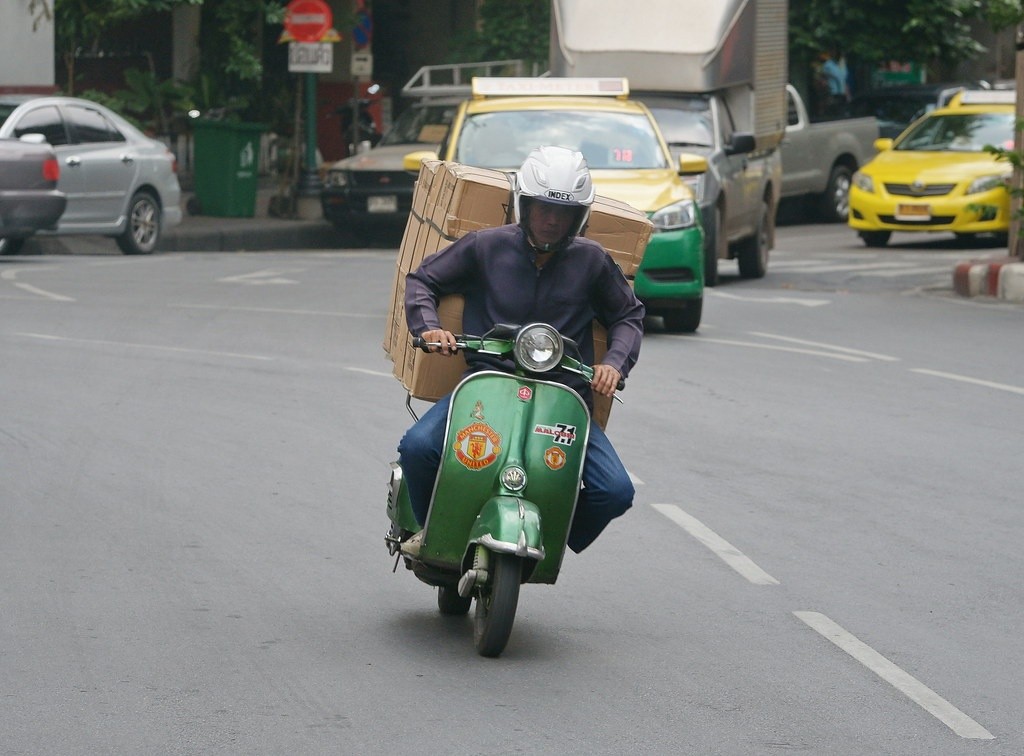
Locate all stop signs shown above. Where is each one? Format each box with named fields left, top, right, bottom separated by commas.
left=284, top=0, right=333, bottom=44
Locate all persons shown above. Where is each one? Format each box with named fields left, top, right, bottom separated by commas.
left=397, top=145, right=645, bottom=554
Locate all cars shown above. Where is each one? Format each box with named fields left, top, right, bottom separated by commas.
left=320, top=59, right=536, bottom=248
left=0, top=141, right=67, bottom=240
left=847, top=91, right=1017, bottom=247
left=0, top=93, right=185, bottom=256
left=404, top=75, right=708, bottom=333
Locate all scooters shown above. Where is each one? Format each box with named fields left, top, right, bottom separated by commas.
left=383, top=321, right=625, bottom=659
left=328, top=83, right=384, bottom=153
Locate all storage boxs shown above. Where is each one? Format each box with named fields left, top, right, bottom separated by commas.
left=382, top=159, right=653, bottom=436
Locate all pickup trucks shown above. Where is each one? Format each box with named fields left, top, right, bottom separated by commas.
left=779, top=83, right=880, bottom=223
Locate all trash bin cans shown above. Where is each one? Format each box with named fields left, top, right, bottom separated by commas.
left=184, top=116, right=272, bottom=219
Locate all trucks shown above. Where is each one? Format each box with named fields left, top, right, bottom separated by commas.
left=538, top=0, right=790, bottom=286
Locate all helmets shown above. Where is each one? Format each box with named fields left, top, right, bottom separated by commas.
left=513, top=147, right=596, bottom=238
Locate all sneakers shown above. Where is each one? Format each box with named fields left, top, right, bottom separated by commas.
left=398, top=526, right=424, bottom=557
